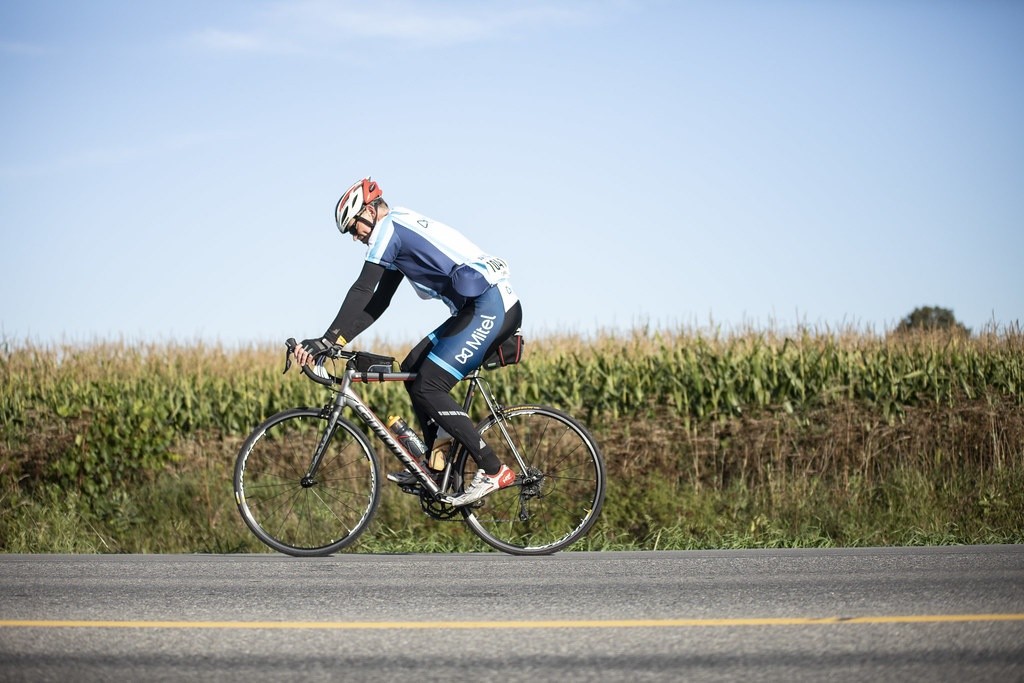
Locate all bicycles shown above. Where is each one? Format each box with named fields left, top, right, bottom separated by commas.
left=233, top=337, right=606, bottom=556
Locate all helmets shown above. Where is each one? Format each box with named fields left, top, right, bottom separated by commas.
left=335, top=176, right=382, bottom=234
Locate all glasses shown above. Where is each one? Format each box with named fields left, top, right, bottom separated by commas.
left=348, top=203, right=377, bottom=235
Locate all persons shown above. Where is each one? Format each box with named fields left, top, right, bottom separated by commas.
left=294, top=176, right=521, bottom=507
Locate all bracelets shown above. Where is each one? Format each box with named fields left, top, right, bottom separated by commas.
left=339, top=335, right=347, bottom=344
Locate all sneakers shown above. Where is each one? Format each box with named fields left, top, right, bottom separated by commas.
left=452, top=463, right=516, bottom=507
left=386, top=459, right=445, bottom=485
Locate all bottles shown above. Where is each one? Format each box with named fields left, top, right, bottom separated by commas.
left=428, top=426, right=453, bottom=471
left=387, top=415, right=429, bottom=458
